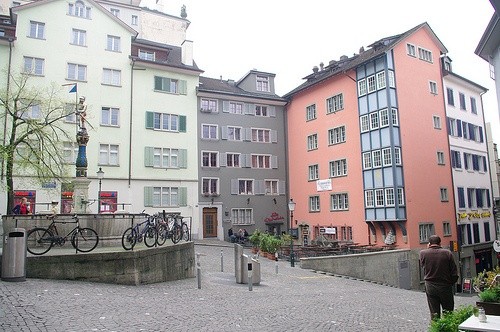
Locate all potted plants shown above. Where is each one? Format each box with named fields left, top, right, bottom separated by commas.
left=249, top=229, right=292, bottom=260
left=476, top=284, right=500, bottom=316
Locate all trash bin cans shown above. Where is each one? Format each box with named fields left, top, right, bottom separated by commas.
left=0, top=228, right=27, bottom=281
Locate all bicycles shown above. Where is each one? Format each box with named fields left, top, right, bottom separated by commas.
left=120, top=210, right=190, bottom=250
left=26, top=212, right=100, bottom=254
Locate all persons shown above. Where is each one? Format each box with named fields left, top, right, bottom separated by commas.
left=13, top=197, right=27, bottom=214
left=228, top=227, right=248, bottom=242
left=75, top=96, right=87, bottom=128
left=420, top=235, right=458, bottom=322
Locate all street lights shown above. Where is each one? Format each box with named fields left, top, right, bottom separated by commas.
left=96, top=167, right=105, bottom=213
left=287, top=197, right=295, bottom=267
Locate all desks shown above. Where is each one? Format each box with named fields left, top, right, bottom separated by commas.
left=458, top=314, right=500, bottom=332
left=276, top=241, right=398, bottom=262
